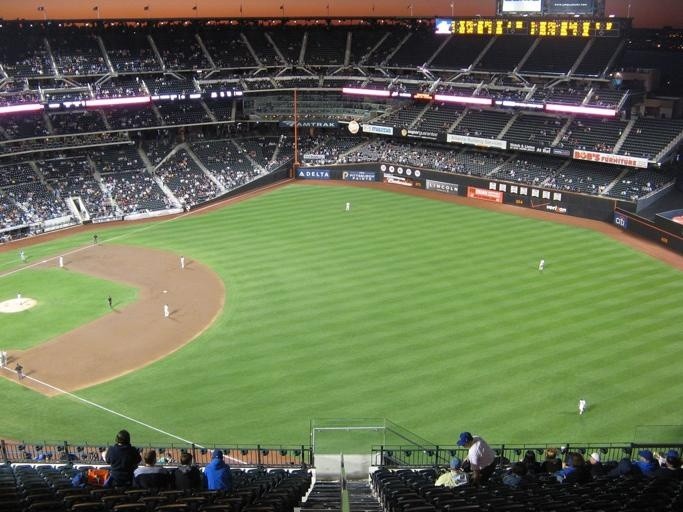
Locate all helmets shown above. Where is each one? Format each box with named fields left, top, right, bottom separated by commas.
left=117, top=430, right=130, bottom=443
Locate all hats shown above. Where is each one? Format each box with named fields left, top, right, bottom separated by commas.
left=450, top=457, right=460, bottom=469
left=665, top=450, right=678, bottom=457
left=457, top=432, right=471, bottom=446
left=638, top=450, right=652, bottom=459
left=588, top=453, right=599, bottom=462
left=213, top=449, right=222, bottom=458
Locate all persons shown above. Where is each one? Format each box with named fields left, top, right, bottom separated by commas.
left=2, top=350, right=8, bottom=367
left=59, top=256, right=64, bottom=268
left=0, top=205, right=192, bottom=248
left=171, top=452, right=204, bottom=491
left=345, top=203, right=349, bottom=212
left=107, top=295, right=112, bottom=307
left=538, top=259, right=545, bottom=271
left=19, top=250, right=25, bottom=262
left=180, top=256, right=185, bottom=267
left=14, top=362, right=24, bottom=381
left=579, top=397, right=588, bottom=416
left=0, top=354, right=4, bottom=368
left=132, top=449, right=170, bottom=492
left=101, top=428, right=142, bottom=489
left=23, top=447, right=178, bottom=465
left=163, top=303, right=169, bottom=316
left=432, top=428, right=683, bottom=491
left=204, top=449, right=234, bottom=492
left=0, top=17, right=667, bottom=205
